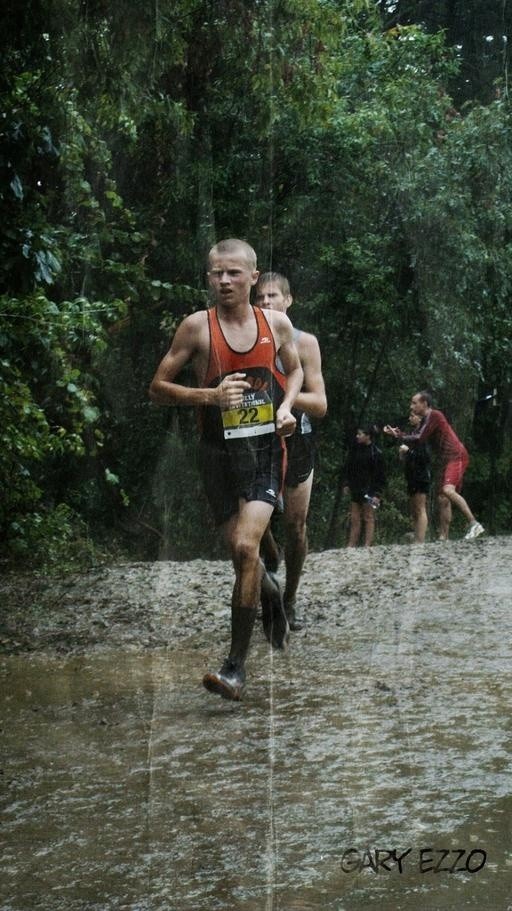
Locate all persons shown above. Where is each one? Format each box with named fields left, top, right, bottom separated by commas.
left=398, top=409, right=432, bottom=550
left=385, top=391, right=486, bottom=544
left=148, top=238, right=305, bottom=700
left=253, top=271, right=329, bottom=631
left=342, top=426, right=387, bottom=548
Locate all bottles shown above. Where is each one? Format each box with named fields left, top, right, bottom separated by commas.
left=364, top=494, right=381, bottom=509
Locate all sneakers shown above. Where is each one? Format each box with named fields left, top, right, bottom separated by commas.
left=465, top=523, right=484, bottom=539
left=265, top=571, right=303, bottom=647
left=203, top=659, right=247, bottom=701
